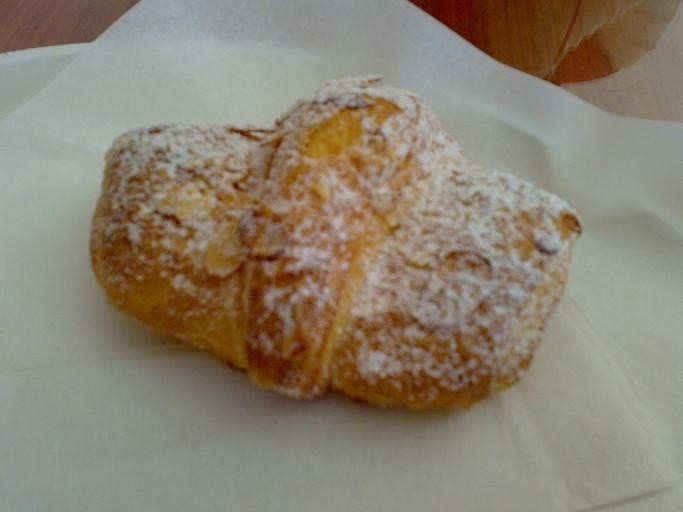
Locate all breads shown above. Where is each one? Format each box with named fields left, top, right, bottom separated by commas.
left=89, top=72, right=584, bottom=412
